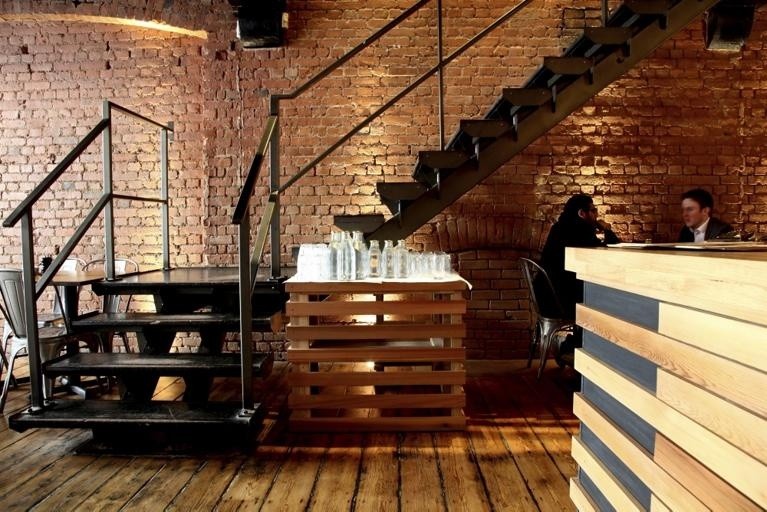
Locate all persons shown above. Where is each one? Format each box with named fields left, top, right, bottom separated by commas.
left=532, top=194, right=621, bottom=365
left=678, top=189, right=731, bottom=242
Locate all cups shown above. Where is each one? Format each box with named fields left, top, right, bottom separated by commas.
left=409, top=249, right=452, bottom=279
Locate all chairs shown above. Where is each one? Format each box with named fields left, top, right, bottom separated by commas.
left=518, top=256, right=577, bottom=380
left=1, top=256, right=139, bottom=410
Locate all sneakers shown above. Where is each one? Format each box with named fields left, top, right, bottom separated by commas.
left=558, top=334, right=575, bottom=370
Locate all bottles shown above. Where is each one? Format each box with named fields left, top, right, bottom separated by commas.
left=329, top=230, right=409, bottom=281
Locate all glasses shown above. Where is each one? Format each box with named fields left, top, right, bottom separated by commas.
left=590, top=208, right=598, bottom=213
